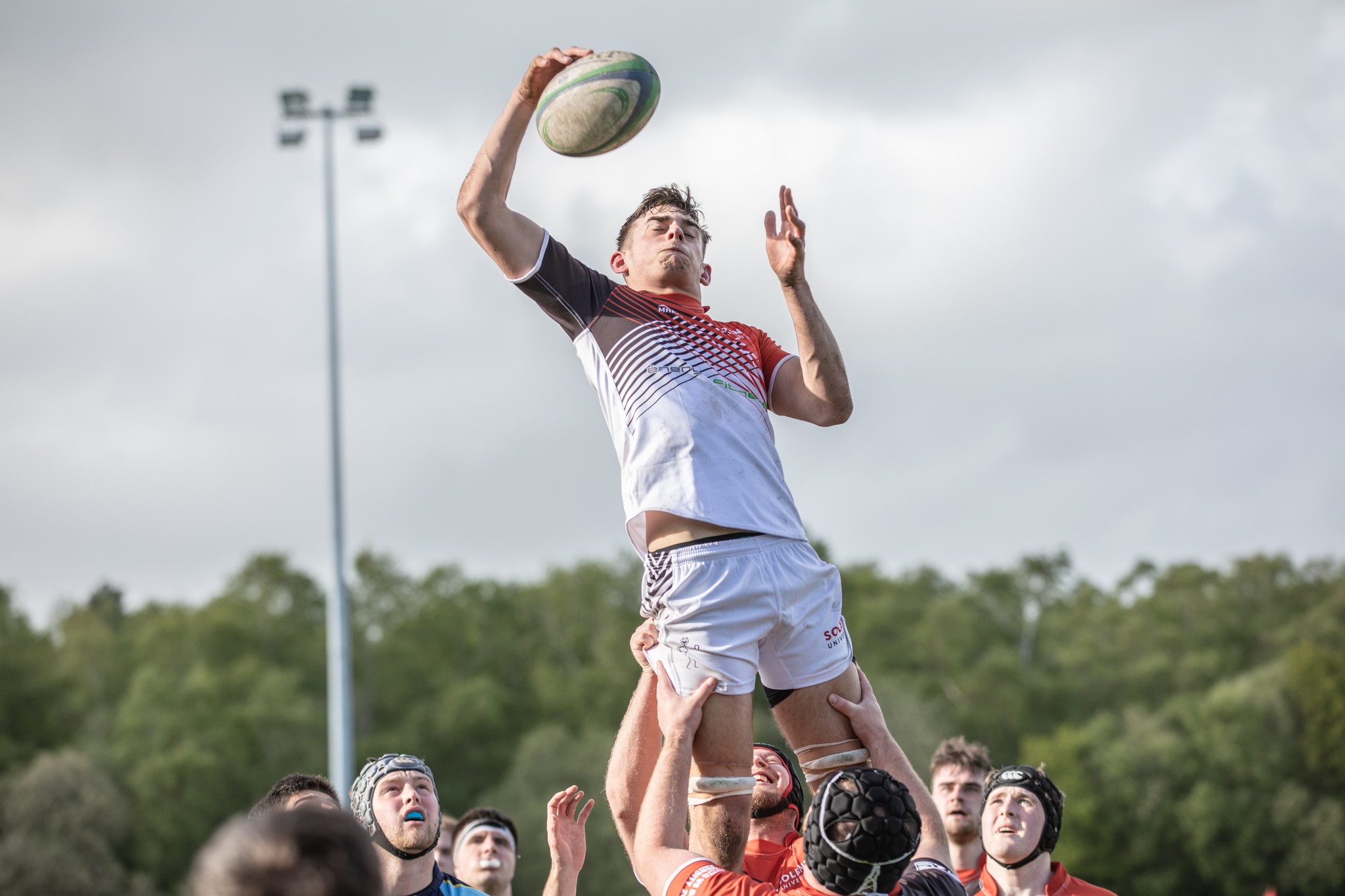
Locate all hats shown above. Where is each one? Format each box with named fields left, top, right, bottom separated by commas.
left=979, top=766, right=1063, bottom=853
left=803, top=768, right=922, bottom=896
left=351, top=753, right=442, bottom=841
left=749, top=742, right=803, bottom=835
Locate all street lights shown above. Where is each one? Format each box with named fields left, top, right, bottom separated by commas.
left=276, top=86, right=390, bottom=821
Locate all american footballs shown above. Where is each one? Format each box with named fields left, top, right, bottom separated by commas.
left=536, top=50, right=661, bottom=157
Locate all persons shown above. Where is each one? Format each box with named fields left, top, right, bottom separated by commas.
left=956, top=765, right=1116, bottom=896
left=457, top=44, right=873, bottom=870
left=605, top=619, right=965, bottom=896
left=928, top=738, right=998, bottom=885
left=0, top=752, right=595, bottom=896
